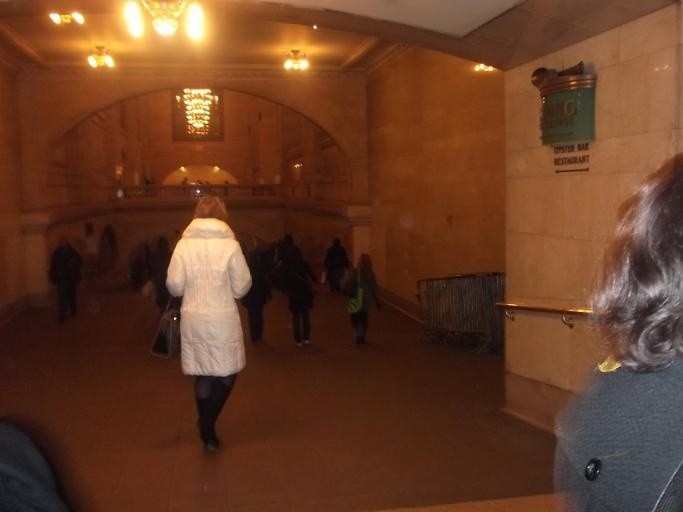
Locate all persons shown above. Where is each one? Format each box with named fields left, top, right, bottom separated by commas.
left=323, top=237, right=352, bottom=295
left=1, top=419, right=75, bottom=512
left=241, top=234, right=319, bottom=347
left=79, top=223, right=117, bottom=276
left=547, top=150, right=681, bottom=512
left=345, top=253, right=384, bottom=346
left=162, top=193, right=254, bottom=456
left=134, top=231, right=182, bottom=348
left=47, top=235, right=84, bottom=322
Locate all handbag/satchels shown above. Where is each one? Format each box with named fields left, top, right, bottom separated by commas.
left=150, top=294, right=181, bottom=360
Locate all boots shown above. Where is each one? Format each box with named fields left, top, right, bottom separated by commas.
left=197, top=415, right=219, bottom=452
